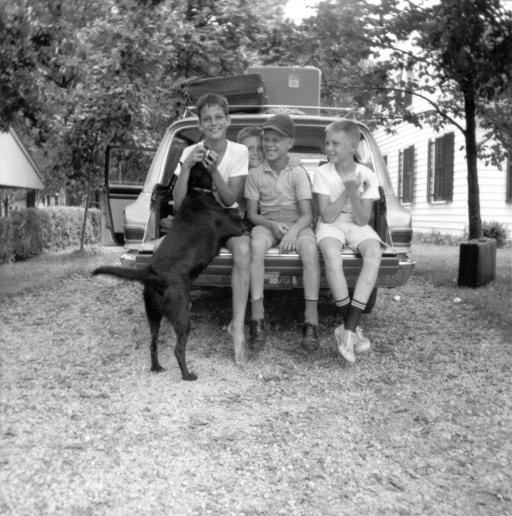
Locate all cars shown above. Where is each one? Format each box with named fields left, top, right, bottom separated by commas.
left=104, top=104, right=417, bottom=289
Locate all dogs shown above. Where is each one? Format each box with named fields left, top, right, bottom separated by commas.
left=89, top=146, right=256, bottom=382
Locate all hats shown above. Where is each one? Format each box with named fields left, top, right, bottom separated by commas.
left=257, top=115, right=295, bottom=140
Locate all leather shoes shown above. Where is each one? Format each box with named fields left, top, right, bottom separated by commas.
left=248, top=319, right=266, bottom=349
left=302, top=323, right=321, bottom=350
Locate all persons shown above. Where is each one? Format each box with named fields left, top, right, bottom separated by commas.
left=309, top=118, right=387, bottom=365
left=243, top=111, right=322, bottom=355
left=236, top=124, right=303, bottom=232
left=152, top=89, right=252, bottom=366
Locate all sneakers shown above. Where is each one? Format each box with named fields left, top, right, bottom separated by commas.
left=334, top=325, right=357, bottom=362
left=354, top=326, right=371, bottom=353
